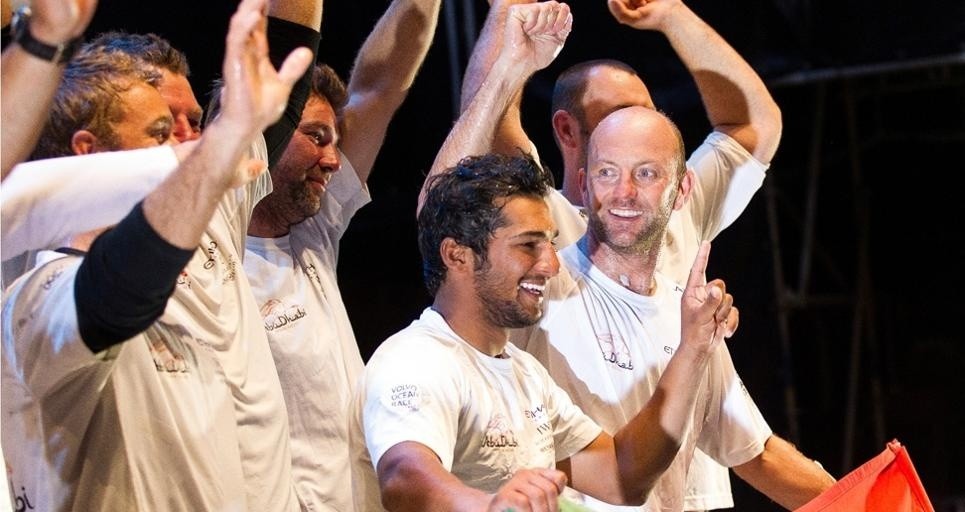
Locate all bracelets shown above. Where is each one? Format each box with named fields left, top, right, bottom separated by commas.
left=9, top=2, right=83, bottom=65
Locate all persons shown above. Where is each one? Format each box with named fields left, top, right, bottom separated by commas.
left=357, top=144, right=741, bottom=512
left=0, top=0, right=101, bottom=179
left=0, top=138, right=270, bottom=262
left=242, top=0, right=445, bottom=512
left=73, top=0, right=327, bottom=511
left=417, top=0, right=840, bottom=512
left=0, top=0, right=313, bottom=511
left=457, top=0, right=785, bottom=285
